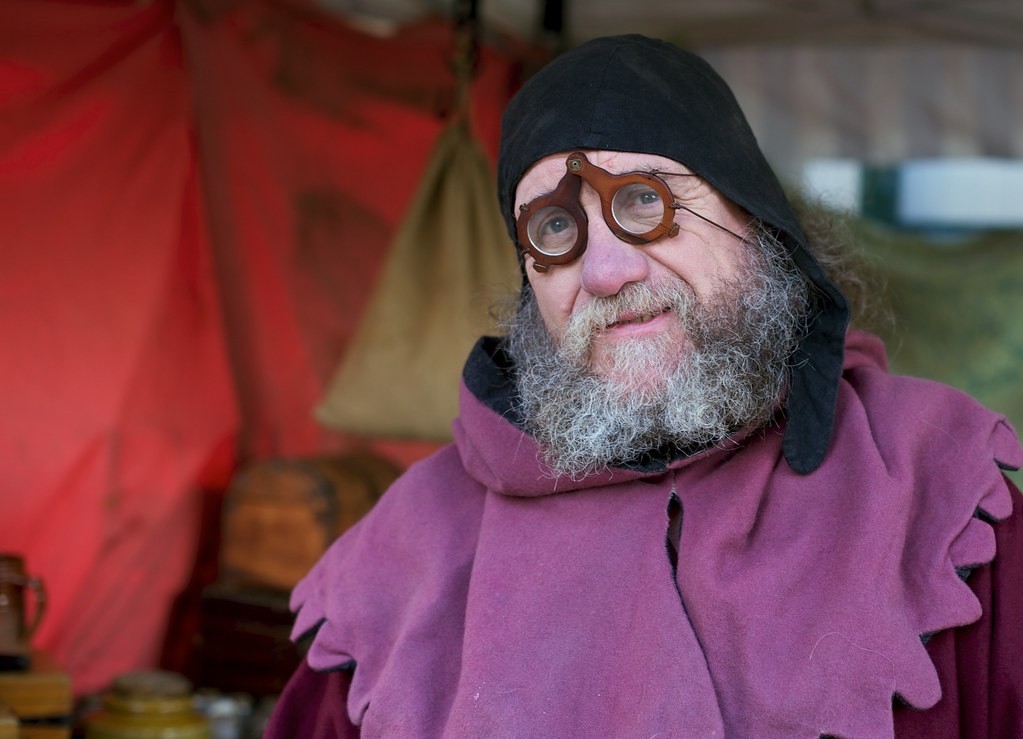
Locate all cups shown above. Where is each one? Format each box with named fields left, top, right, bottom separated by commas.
left=1, top=557, right=46, bottom=671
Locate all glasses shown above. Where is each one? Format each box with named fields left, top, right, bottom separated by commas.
left=515, top=152, right=762, bottom=273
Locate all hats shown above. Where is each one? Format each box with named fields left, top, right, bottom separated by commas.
left=497, top=35, right=852, bottom=476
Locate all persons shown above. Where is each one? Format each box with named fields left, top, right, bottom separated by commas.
left=264, top=35, right=1023, bottom=739
